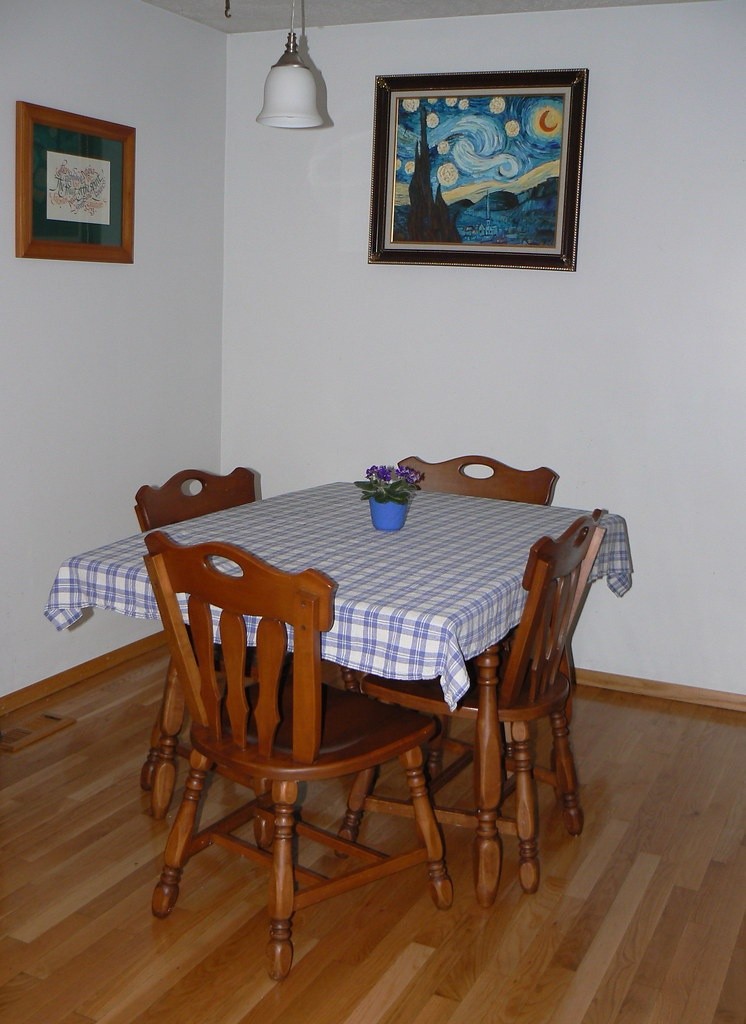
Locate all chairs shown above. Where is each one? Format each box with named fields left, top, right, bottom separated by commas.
left=332, top=454, right=606, bottom=892
left=132, top=465, right=273, bottom=800
left=141, top=529, right=455, bottom=984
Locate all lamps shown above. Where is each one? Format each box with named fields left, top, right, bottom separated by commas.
left=255, top=0, right=324, bottom=129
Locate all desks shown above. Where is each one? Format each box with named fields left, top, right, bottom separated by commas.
left=43, top=476, right=637, bottom=909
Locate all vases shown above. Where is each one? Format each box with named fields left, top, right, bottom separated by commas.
left=367, top=496, right=408, bottom=531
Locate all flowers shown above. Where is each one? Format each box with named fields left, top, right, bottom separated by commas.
left=353, top=464, right=424, bottom=505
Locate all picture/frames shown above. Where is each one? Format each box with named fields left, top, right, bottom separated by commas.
left=367, top=68, right=591, bottom=271
left=15, top=100, right=134, bottom=265
